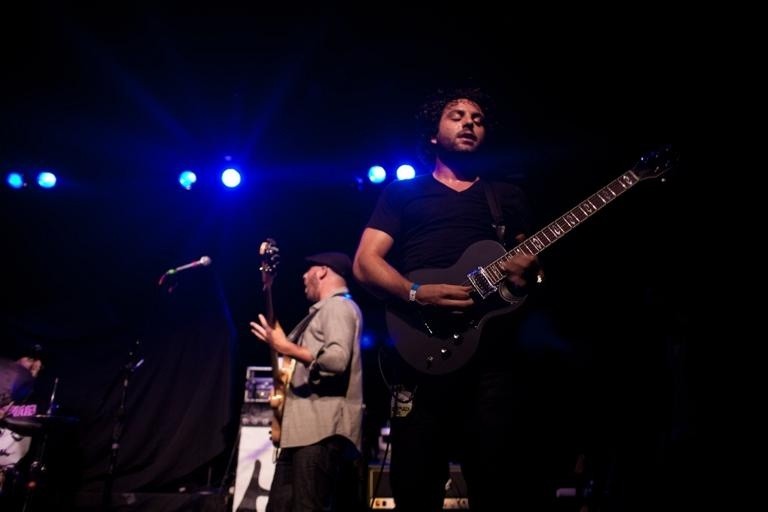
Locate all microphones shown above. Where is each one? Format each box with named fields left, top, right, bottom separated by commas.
left=176, top=256, right=211, bottom=272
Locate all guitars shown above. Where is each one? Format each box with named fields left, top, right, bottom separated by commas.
left=386, top=153, right=670, bottom=374
left=259, top=237, right=289, bottom=448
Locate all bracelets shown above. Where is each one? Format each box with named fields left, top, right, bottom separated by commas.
left=407, top=280, right=421, bottom=303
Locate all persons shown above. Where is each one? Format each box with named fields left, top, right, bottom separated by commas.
left=247, top=249, right=366, bottom=512
left=0, top=341, right=46, bottom=415
left=345, top=82, right=547, bottom=510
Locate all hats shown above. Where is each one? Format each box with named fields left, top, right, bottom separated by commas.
left=306, top=252, right=351, bottom=280
